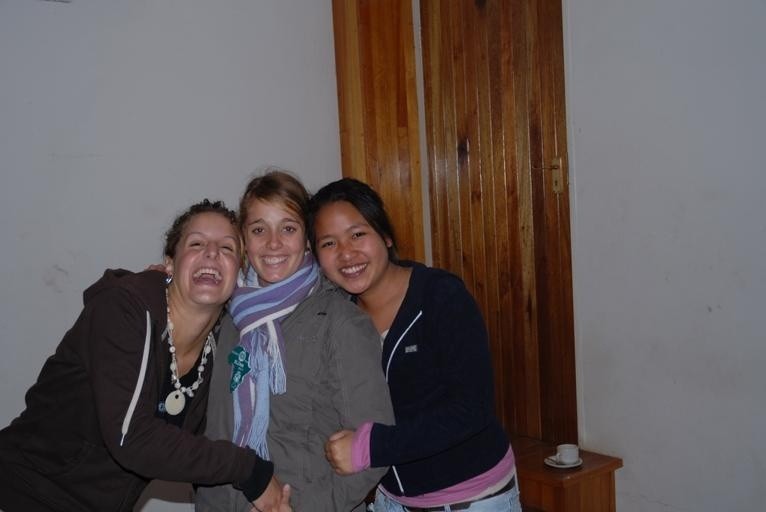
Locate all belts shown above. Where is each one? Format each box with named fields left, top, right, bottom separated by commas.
left=401, top=477, right=516, bottom=512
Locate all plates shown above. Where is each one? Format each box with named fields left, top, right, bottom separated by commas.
left=544, top=455, right=583, bottom=469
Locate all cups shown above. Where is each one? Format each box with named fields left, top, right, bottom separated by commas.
left=556, top=443, right=580, bottom=464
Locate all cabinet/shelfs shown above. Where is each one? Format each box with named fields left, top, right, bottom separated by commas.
left=514, top=446, right=623, bottom=512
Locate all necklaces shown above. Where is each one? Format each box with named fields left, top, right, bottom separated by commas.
left=163, top=284, right=213, bottom=417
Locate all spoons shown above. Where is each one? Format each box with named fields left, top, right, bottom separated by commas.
left=544, top=456, right=565, bottom=466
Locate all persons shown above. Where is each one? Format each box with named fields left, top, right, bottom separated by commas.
left=1, top=198, right=282, bottom=511
left=144, top=166, right=396, bottom=511
left=306, top=178, right=524, bottom=511
